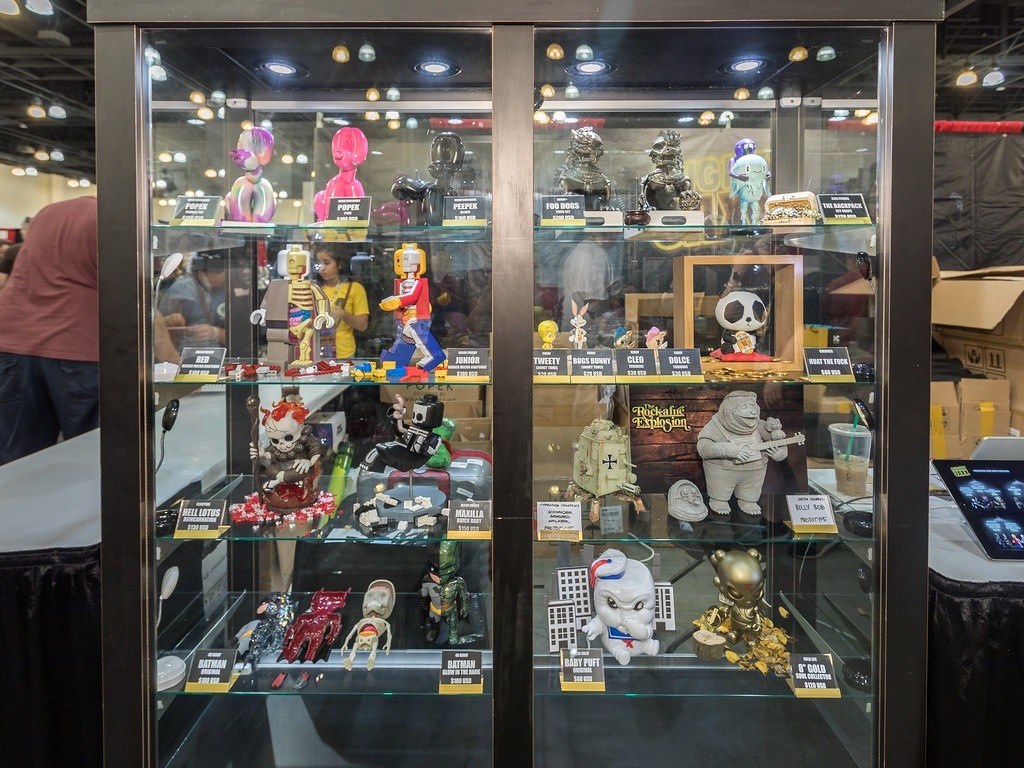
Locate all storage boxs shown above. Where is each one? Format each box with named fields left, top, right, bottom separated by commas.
left=830, top=256, right=1024, bottom=459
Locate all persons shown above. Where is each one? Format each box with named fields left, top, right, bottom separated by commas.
left=151, top=202, right=786, bottom=376
left=0, top=194, right=101, bottom=466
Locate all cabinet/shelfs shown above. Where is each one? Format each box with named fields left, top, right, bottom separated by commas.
left=85, top=0, right=947, bottom=768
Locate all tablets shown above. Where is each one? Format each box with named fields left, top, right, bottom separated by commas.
left=931, top=458, right=1024, bottom=562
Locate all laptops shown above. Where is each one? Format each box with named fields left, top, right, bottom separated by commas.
left=970, top=437, right=1024, bottom=461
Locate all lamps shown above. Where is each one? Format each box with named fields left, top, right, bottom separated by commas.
left=956, top=50, right=976, bottom=86
left=0, top=0, right=877, bottom=199
left=982, top=47, right=1005, bottom=87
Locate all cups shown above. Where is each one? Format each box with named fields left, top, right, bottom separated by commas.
left=827, top=422, right=872, bottom=496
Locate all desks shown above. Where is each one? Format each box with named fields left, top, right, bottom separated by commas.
left=807, top=462, right=1024, bottom=768
left=0, top=360, right=371, bottom=768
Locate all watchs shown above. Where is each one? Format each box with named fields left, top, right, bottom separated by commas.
left=730, top=271, right=743, bottom=281
